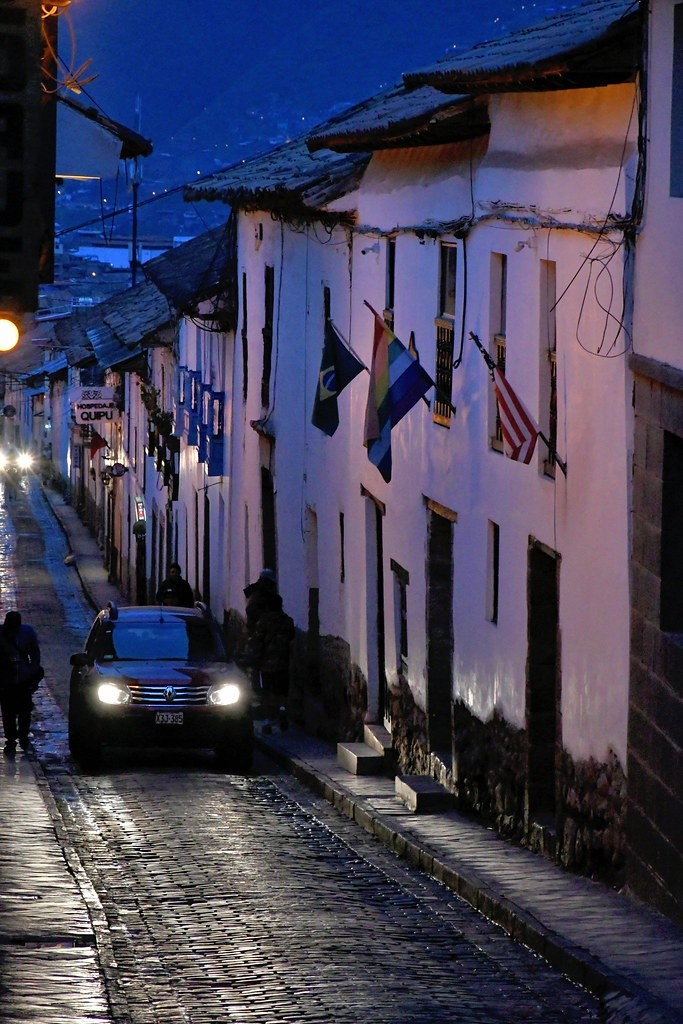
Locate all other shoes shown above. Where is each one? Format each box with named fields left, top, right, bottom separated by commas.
left=2, top=738, right=28, bottom=755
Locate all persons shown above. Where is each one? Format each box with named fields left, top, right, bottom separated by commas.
left=244, top=568, right=277, bottom=630
left=155, top=563, right=194, bottom=608
left=248, top=595, right=291, bottom=727
left=0, top=611, right=40, bottom=759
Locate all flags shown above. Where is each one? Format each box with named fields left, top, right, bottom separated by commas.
left=310, top=317, right=364, bottom=437
left=493, top=369, right=540, bottom=467
left=90, top=431, right=107, bottom=460
left=364, top=316, right=434, bottom=485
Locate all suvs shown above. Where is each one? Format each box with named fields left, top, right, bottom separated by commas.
left=64, top=601, right=258, bottom=771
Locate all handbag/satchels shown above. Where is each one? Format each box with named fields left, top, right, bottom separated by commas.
left=28, top=663, right=44, bottom=694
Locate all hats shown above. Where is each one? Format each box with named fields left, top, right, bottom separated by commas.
left=4, top=611, right=22, bottom=629
left=260, top=569, right=280, bottom=583
